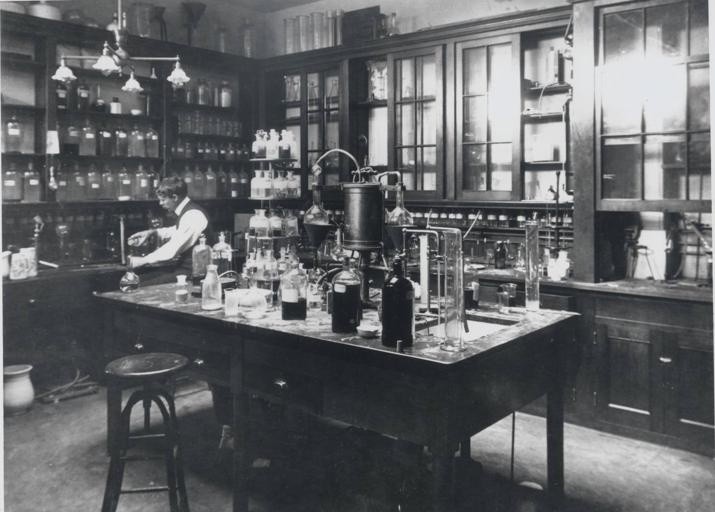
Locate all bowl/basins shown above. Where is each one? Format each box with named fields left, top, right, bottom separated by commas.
left=356, top=325, right=378, bottom=337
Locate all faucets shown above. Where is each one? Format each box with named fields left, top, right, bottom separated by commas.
left=311, top=149, right=361, bottom=183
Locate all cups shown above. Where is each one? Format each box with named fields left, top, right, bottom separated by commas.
left=176, top=290, right=189, bottom=303
left=176, top=275, right=187, bottom=284
left=496, top=283, right=516, bottom=314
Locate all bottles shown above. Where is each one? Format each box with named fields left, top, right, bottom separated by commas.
left=407, top=212, right=573, bottom=283
left=381, top=260, right=415, bottom=348
left=463, top=257, right=480, bottom=311
left=128, top=238, right=148, bottom=247
left=521, top=220, right=544, bottom=316
left=440, top=229, right=468, bottom=352
left=2, top=246, right=38, bottom=280
left=331, top=257, right=361, bottom=333
left=192, top=183, right=331, bottom=321
left=386, top=182, right=414, bottom=226
left=120, top=255, right=140, bottom=294
left=633, top=245, right=655, bottom=287
left=0, top=1, right=397, bottom=202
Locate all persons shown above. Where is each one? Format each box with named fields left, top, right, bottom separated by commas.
left=127, top=174, right=219, bottom=272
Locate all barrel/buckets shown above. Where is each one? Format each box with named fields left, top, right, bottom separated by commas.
left=343, top=183, right=384, bottom=251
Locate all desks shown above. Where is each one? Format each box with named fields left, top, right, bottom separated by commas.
left=90, top=279, right=583, bottom=512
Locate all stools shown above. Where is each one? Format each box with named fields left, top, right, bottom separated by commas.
left=101, top=352, right=192, bottom=511
